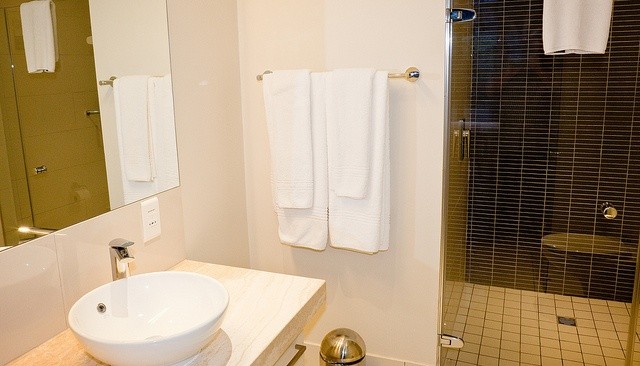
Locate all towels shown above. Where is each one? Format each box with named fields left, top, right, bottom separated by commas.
left=542, top=0, right=613, bottom=54
left=327, top=72, right=389, bottom=253
left=20, top=1, right=61, bottom=73
left=266, top=70, right=328, bottom=251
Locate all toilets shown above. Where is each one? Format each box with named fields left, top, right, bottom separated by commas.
left=541, top=234, right=635, bottom=300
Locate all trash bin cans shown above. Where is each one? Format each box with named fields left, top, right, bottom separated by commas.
left=317, top=327, right=366, bottom=366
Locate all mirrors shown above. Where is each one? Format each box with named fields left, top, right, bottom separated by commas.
left=1, top=0, right=181, bottom=254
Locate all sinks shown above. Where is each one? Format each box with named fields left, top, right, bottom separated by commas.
left=67, top=271, right=229, bottom=343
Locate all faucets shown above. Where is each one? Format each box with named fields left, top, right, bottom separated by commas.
left=109, top=238, right=135, bottom=281
left=18, top=226, right=58, bottom=245
